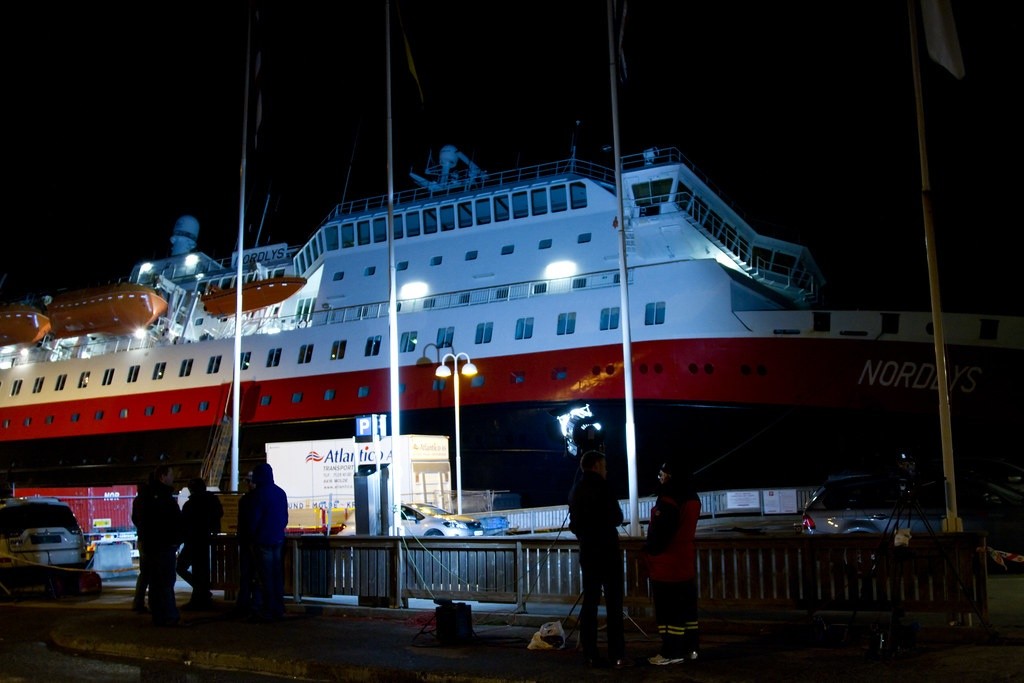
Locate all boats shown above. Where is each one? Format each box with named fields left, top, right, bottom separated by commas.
left=0, top=146, right=1024, bottom=507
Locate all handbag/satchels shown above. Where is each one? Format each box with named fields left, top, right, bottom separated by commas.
left=540, top=621, right=566, bottom=650
left=527, top=633, right=557, bottom=650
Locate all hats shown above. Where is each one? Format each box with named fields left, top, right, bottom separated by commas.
left=245, top=471, right=253, bottom=479
left=661, top=461, right=683, bottom=475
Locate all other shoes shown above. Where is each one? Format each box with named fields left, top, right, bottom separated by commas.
left=153, top=614, right=182, bottom=625
left=133, top=606, right=148, bottom=613
left=586, top=657, right=598, bottom=666
left=613, top=659, right=625, bottom=669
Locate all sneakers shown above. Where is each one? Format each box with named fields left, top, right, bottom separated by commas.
left=687, top=650, right=698, bottom=660
left=648, top=654, right=686, bottom=666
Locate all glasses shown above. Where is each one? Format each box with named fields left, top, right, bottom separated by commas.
left=658, top=473, right=666, bottom=477
left=245, top=479, right=256, bottom=484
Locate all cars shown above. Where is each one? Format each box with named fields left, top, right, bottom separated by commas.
left=0, top=495, right=87, bottom=593
left=338, top=502, right=485, bottom=537
left=800, top=469, right=1023, bottom=572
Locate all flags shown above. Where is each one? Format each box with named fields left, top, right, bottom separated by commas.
left=923, top=0, right=966, bottom=80
left=392, top=2, right=425, bottom=111
left=615, top=0, right=629, bottom=82
left=251, top=8, right=265, bottom=149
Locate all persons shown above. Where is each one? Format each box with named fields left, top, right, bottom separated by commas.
left=641, top=462, right=703, bottom=664
left=131, top=480, right=151, bottom=610
left=176, top=478, right=222, bottom=609
left=568, top=449, right=630, bottom=668
left=142, top=465, right=191, bottom=627
left=229, top=462, right=289, bottom=618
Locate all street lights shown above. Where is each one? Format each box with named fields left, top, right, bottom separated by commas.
left=435, top=352, right=479, bottom=516
